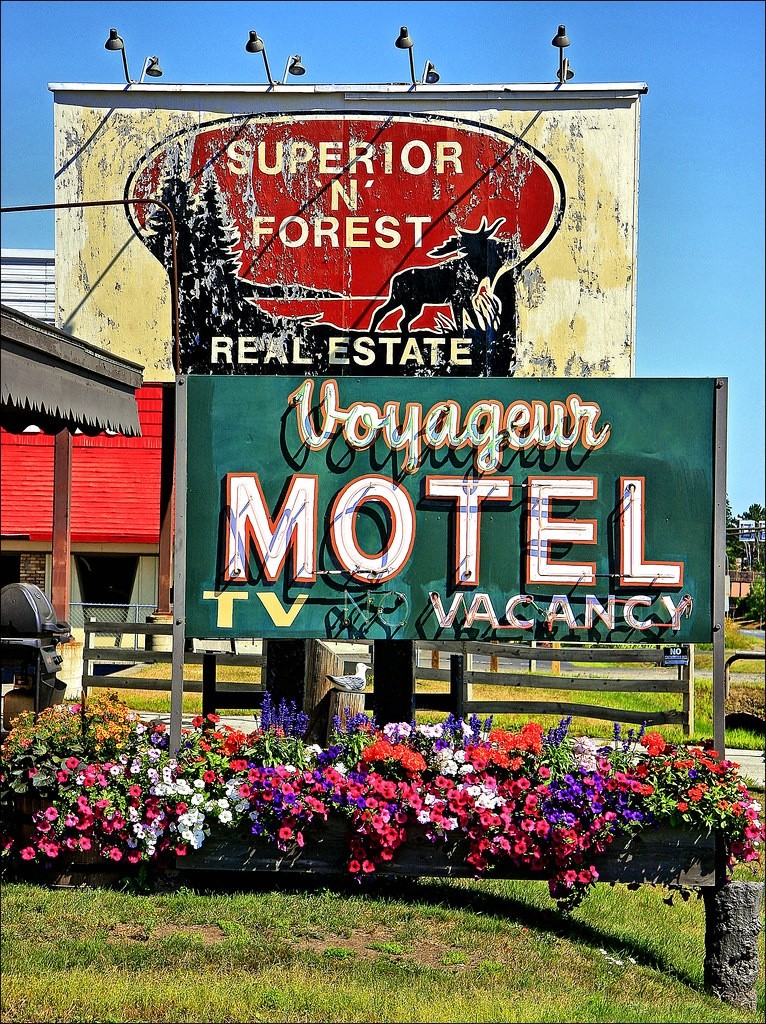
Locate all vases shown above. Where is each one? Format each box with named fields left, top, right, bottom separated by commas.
left=16, top=795, right=105, bottom=865
left=174, top=822, right=722, bottom=877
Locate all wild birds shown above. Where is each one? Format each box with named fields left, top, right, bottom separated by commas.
left=325, top=662, right=372, bottom=692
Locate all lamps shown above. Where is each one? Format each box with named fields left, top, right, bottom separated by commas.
left=551, top=24, right=577, bottom=83
left=283, top=51, right=306, bottom=84
left=104, top=26, right=134, bottom=84
left=424, top=59, right=443, bottom=84
left=393, top=24, right=418, bottom=86
left=241, top=28, right=280, bottom=87
left=141, top=54, right=166, bottom=86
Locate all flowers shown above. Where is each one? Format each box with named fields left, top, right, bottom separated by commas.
left=0, top=695, right=766, bottom=877
